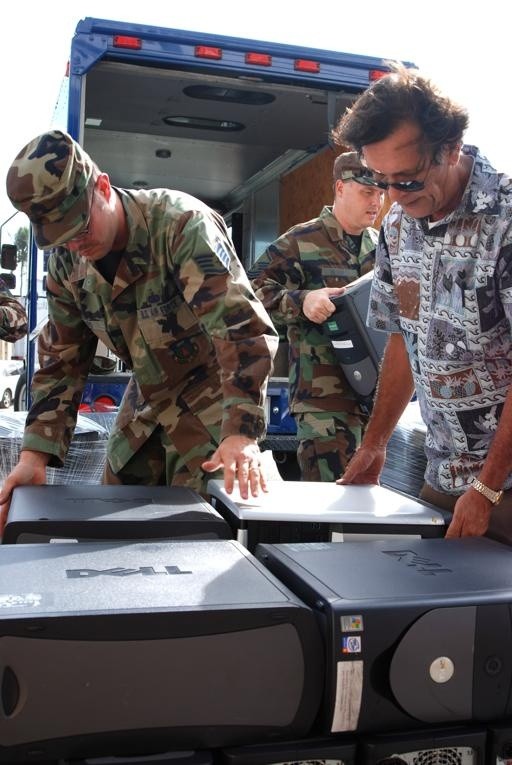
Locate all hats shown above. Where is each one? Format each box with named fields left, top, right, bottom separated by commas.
left=333, top=152, right=379, bottom=186
left=7, top=130, right=94, bottom=251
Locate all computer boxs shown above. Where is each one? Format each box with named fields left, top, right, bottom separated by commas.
left=323, top=269, right=388, bottom=416
left=0, top=480, right=512, bottom=765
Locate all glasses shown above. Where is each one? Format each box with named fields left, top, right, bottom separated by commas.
left=360, top=157, right=433, bottom=192
left=56, top=190, right=94, bottom=247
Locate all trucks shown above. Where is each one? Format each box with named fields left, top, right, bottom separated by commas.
left=2, top=14, right=422, bottom=465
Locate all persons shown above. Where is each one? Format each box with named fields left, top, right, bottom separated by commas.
left=0, top=129, right=280, bottom=536
left=0, top=278, right=28, bottom=344
left=330, top=58, right=512, bottom=546
left=247, top=152, right=385, bottom=482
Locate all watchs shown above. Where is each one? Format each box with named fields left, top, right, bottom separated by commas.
left=470, top=478, right=504, bottom=507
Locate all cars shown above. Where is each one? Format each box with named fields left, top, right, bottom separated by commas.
left=0, top=357, right=26, bottom=408
